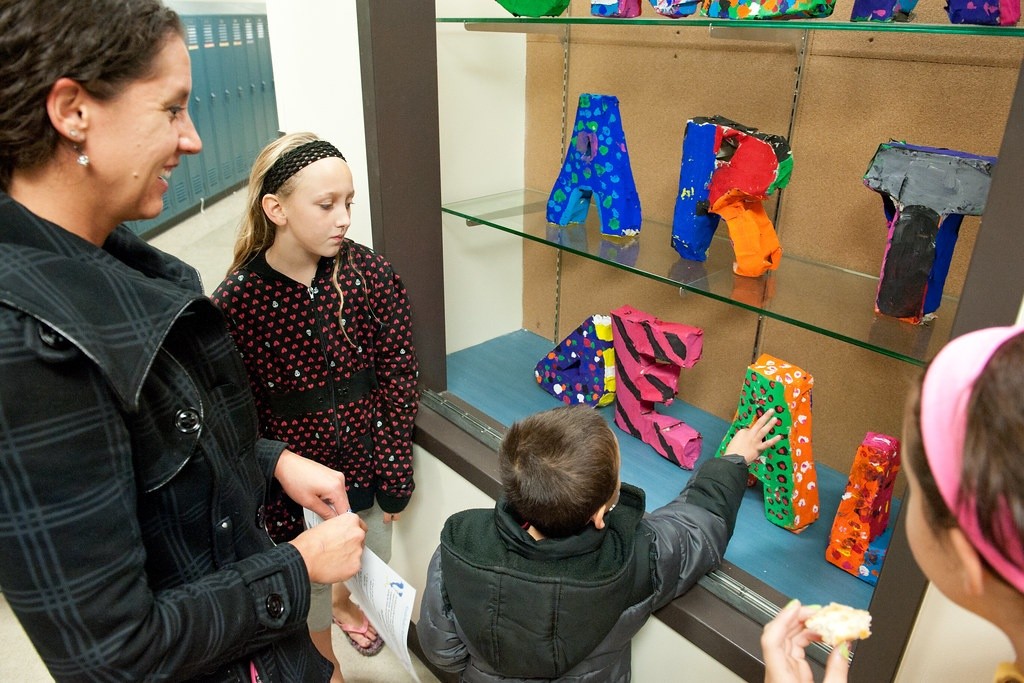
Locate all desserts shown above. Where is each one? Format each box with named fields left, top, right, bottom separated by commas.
left=804, top=602, right=872, bottom=645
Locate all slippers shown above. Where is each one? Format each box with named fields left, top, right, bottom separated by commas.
left=331, top=610, right=384, bottom=656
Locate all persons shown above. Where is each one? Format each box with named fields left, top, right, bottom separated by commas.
left=209, top=128, right=420, bottom=682
left=758, top=327, right=1024, bottom=683
left=415, top=408, right=782, bottom=683
left=1, top=2, right=365, bottom=682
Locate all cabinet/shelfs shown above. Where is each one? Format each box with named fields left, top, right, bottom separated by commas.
left=355, top=1, right=1022, bottom=683
